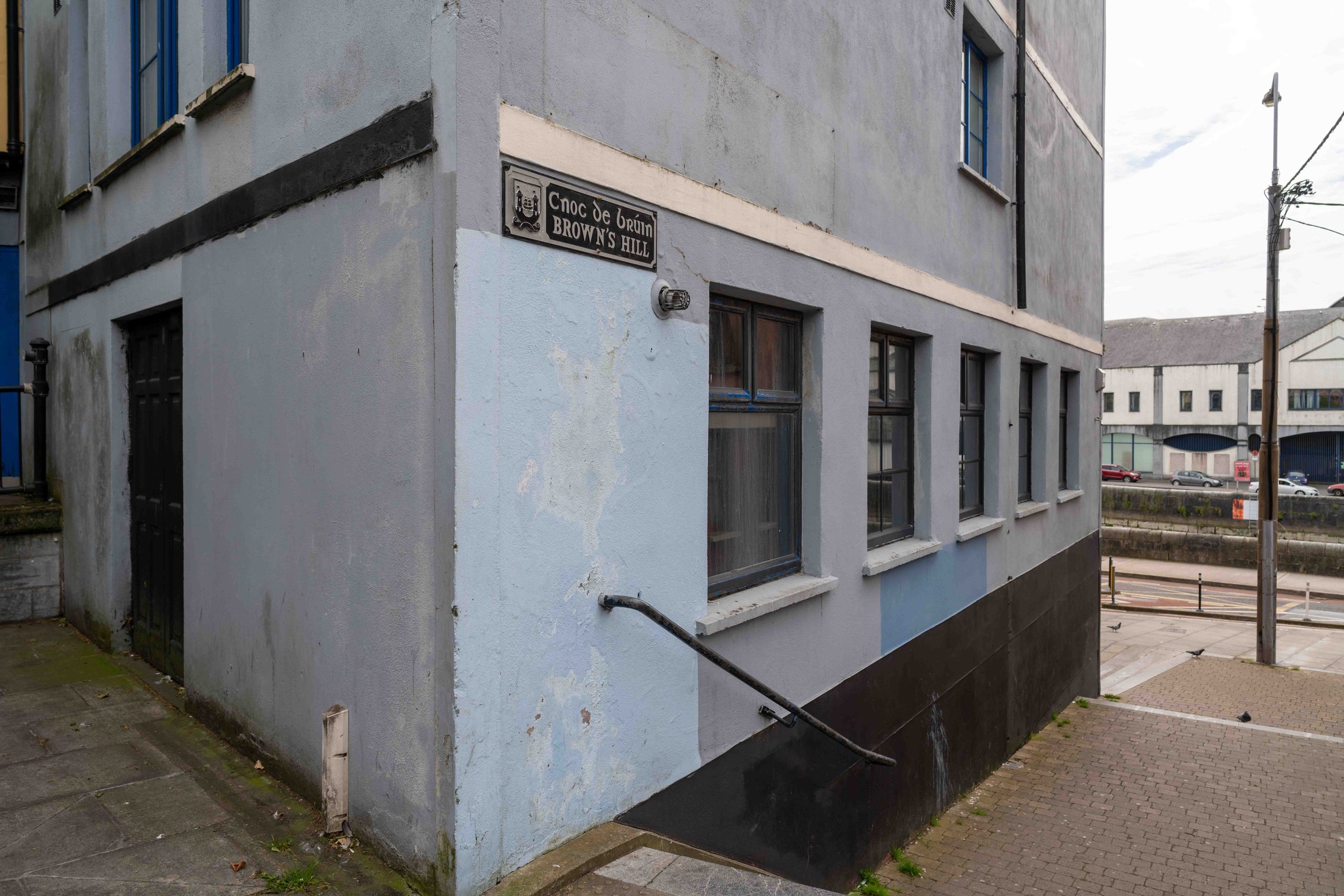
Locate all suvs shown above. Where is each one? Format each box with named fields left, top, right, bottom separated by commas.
left=1102, top=463, right=1142, bottom=483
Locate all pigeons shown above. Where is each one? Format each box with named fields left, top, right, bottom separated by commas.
left=1236, top=711, right=1252, bottom=723
left=1107, top=622, right=1122, bottom=632
left=1186, top=649, right=1205, bottom=658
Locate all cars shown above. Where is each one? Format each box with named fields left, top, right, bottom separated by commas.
left=1247, top=478, right=1320, bottom=496
left=1170, top=470, right=1224, bottom=488
left=1285, top=469, right=1310, bottom=486
left=1327, top=483, right=1344, bottom=496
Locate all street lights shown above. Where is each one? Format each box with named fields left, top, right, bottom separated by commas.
left=1254, top=71, right=1285, bottom=664
